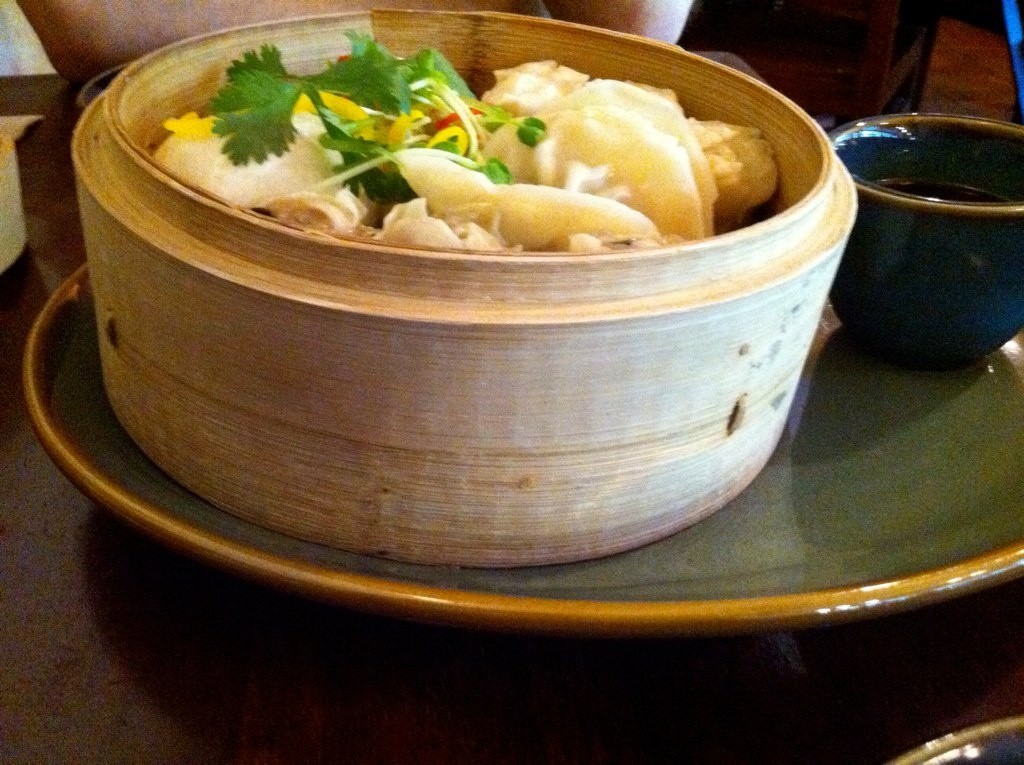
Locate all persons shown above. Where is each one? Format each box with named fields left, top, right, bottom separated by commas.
left=0, top=0, right=696, bottom=311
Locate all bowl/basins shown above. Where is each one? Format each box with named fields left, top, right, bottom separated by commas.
left=72, top=8, right=861, bottom=570
left=826, top=111, right=1024, bottom=374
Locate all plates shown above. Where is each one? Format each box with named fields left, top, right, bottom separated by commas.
left=21, top=263, right=1024, bottom=637
left=887, top=713, right=1024, bottom=765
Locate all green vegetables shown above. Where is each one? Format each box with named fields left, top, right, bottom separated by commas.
left=206, top=27, right=546, bottom=199
